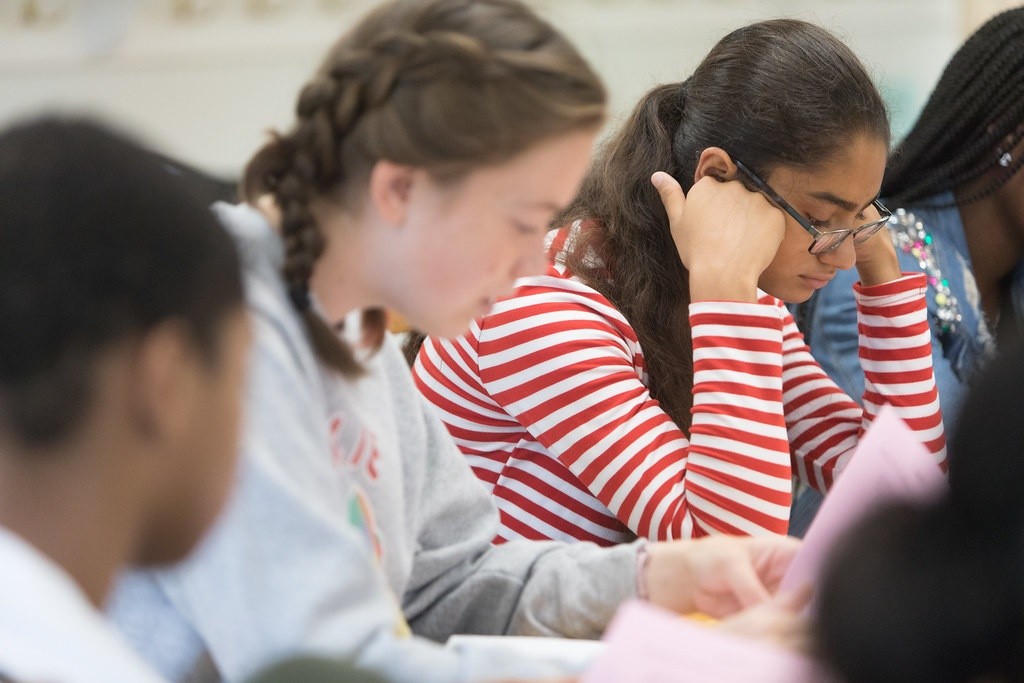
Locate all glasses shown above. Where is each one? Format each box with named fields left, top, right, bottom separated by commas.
left=695, top=150, right=892, bottom=255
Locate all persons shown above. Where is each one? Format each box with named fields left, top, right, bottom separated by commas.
left=789, top=6, right=1024, bottom=538
left=409, top=19, right=951, bottom=548
left=1, top=112, right=253, bottom=683
left=105, top=0, right=813, bottom=683
